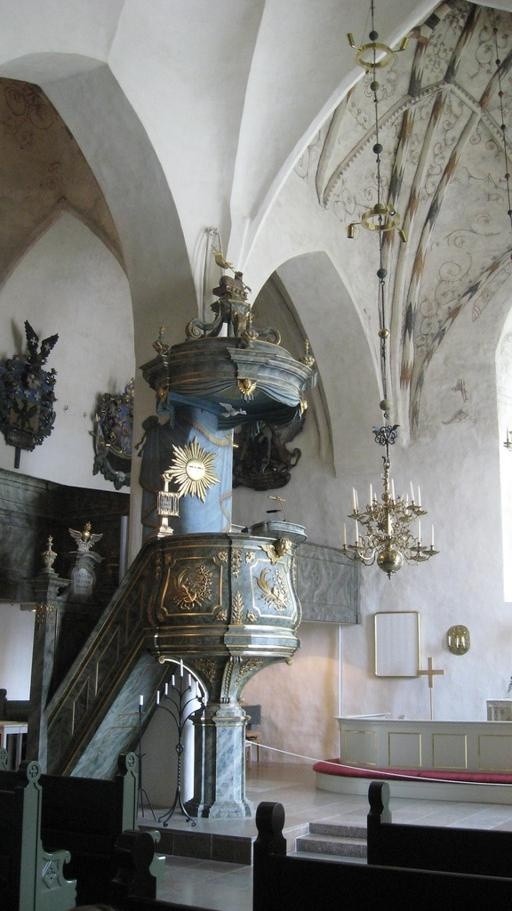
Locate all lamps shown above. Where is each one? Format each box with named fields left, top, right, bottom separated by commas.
left=340, top=0, right=437, bottom=580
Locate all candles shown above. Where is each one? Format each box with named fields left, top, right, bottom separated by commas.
left=188, top=673, right=191, bottom=686
left=172, top=675, right=176, bottom=685
left=204, top=692, right=207, bottom=706
left=165, top=683, right=168, bottom=695
left=180, top=659, right=184, bottom=677
left=196, top=681, right=198, bottom=695
left=156, top=691, right=159, bottom=704
left=139, top=694, right=143, bottom=705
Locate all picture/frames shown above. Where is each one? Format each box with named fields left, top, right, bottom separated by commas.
left=375, top=611, right=420, bottom=678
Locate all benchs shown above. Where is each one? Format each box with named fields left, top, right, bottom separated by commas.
left=367, top=780, right=512, bottom=877
left=1, top=759, right=78, bottom=909
left=70, top=830, right=251, bottom=910
left=254, top=800, right=512, bottom=908
left=41, top=752, right=137, bottom=879
